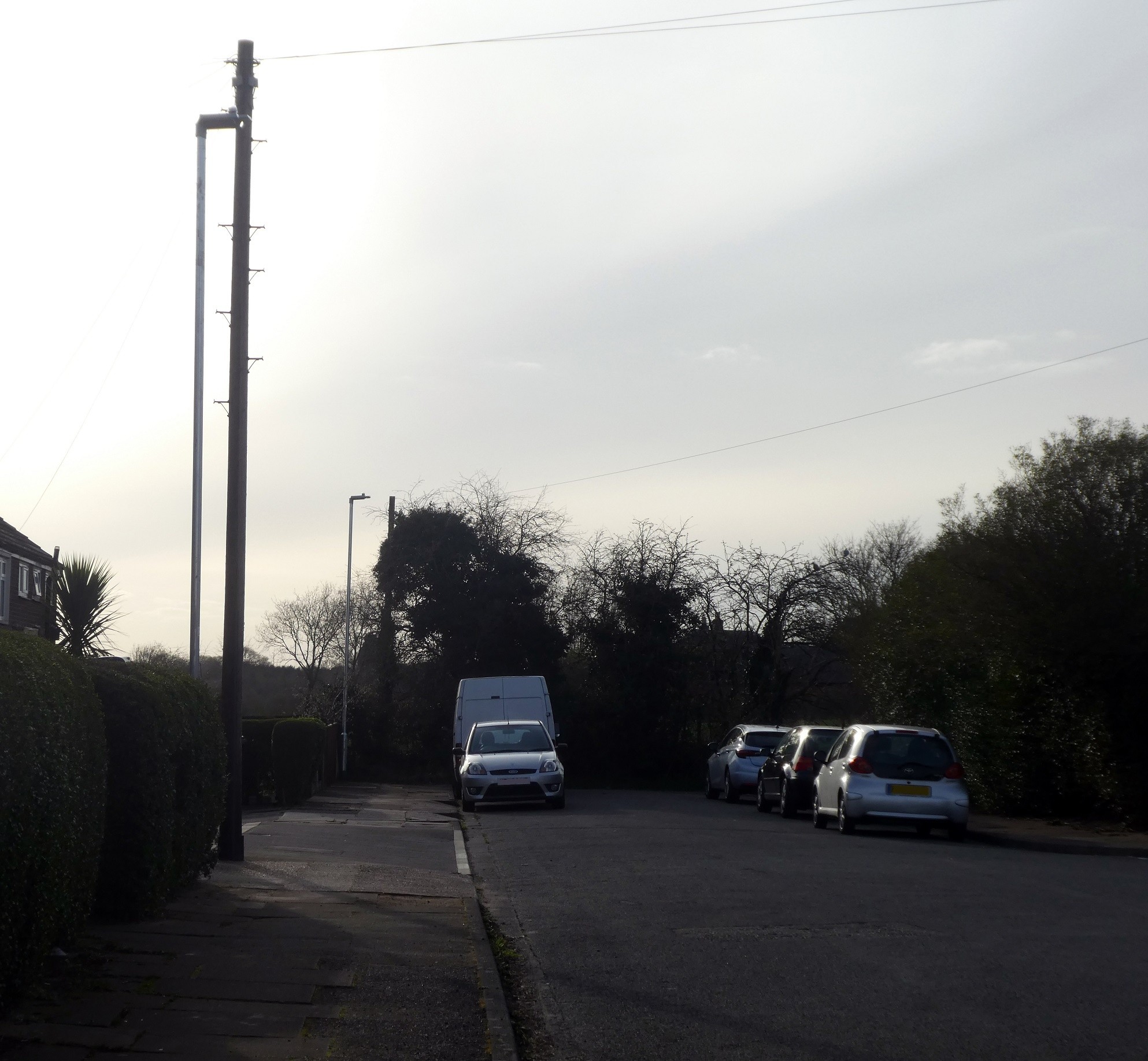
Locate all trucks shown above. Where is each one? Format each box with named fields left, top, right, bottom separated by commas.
left=452, top=675, right=561, bottom=800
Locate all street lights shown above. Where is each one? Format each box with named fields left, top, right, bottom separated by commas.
left=187, top=103, right=253, bottom=682
left=340, top=493, right=373, bottom=781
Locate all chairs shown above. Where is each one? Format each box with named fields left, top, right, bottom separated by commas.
left=519, top=732, right=541, bottom=746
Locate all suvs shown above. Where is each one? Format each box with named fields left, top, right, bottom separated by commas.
left=756, top=725, right=848, bottom=819
left=452, top=719, right=569, bottom=814
left=705, top=723, right=794, bottom=803
left=812, top=724, right=970, bottom=841
